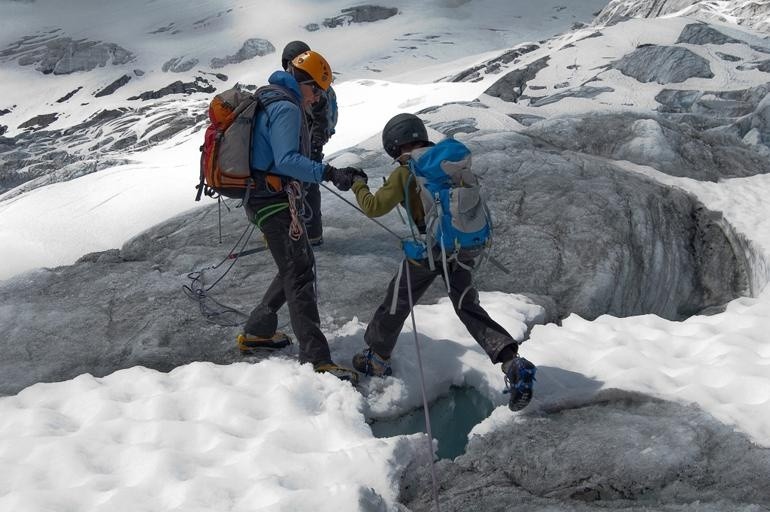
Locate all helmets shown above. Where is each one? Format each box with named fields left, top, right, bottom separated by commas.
left=289, top=52, right=332, bottom=93
left=381, top=113, right=435, bottom=160
left=282, top=41, right=310, bottom=69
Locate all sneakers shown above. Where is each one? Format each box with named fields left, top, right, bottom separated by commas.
left=501, top=356, right=537, bottom=411
left=238, top=331, right=293, bottom=352
left=353, top=353, right=392, bottom=377
left=313, top=362, right=358, bottom=386
left=309, top=234, right=322, bottom=246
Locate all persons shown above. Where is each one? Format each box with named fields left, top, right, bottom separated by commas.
left=354, top=112, right=535, bottom=411
left=234, top=50, right=358, bottom=384
left=281, top=40, right=337, bottom=246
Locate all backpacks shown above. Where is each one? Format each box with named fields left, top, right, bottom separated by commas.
left=401, top=138, right=493, bottom=261
left=196, top=82, right=296, bottom=199
left=321, top=86, right=338, bottom=142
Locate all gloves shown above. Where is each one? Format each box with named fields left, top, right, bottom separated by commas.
left=324, top=163, right=368, bottom=191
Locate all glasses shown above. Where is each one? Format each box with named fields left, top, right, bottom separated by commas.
left=302, top=80, right=324, bottom=97
